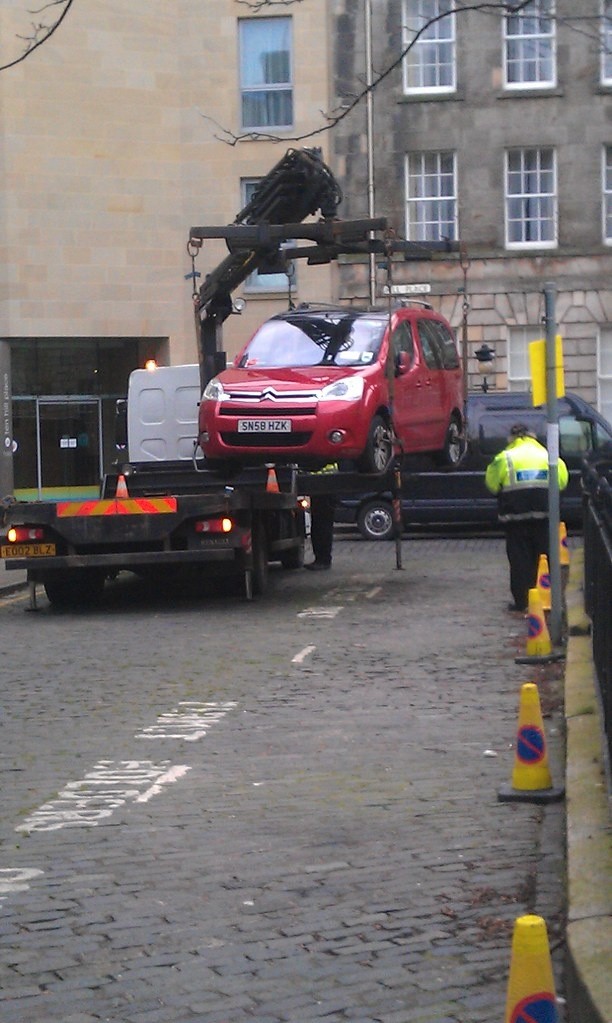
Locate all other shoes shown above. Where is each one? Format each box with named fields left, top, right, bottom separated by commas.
left=507, top=602, right=527, bottom=616
left=303, top=560, right=331, bottom=570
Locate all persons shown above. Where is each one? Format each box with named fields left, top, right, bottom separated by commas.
left=303, top=463, right=339, bottom=570
left=486, top=424, right=568, bottom=612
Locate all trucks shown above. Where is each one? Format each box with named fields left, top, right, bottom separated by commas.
left=0, top=143, right=469, bottom=612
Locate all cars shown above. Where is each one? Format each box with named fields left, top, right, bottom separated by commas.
left=199, top=300, right=467, bottom=475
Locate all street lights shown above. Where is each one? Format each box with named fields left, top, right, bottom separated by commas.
left=473, top=344, right=496, bottom=393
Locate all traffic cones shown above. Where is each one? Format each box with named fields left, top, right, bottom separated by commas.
left=535, top=554, right=554, bottom=612
left=516, top=588, right=563, bottom=663
left=557, top=520, right=568, bottom=567
left=506, top=915, right=557, bottom=1023
left=113, top=474, right=131, bottom=499
left=496, top=683, right=566, bottom=802
left=264, top=468, right=278, bottom=491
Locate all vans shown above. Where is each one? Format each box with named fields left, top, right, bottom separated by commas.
left=336, top=392, right=612, bottom=539
